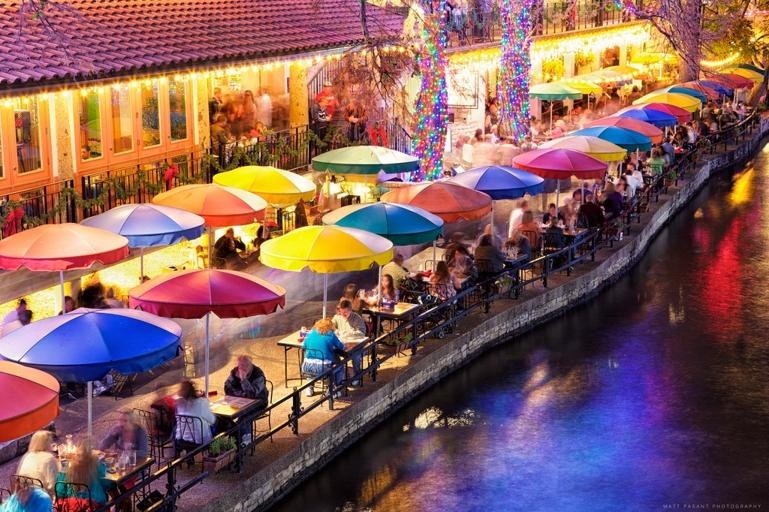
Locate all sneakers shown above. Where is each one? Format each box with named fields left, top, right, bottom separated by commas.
left=306, top=388, right=314, bottom=396
left=352, top=380, right=359, bottom=385
left=332, top=392, right=341, bottom=398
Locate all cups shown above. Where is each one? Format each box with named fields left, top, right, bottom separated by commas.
left=127, top=449, right=137, bottom=466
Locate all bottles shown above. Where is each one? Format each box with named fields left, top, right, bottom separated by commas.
left=105, top=457, right=112, bottom=472
left=66, top=440, right=73, bottom=449
left=118, top=456, right=126, bottom=470
left=573, top=220, right=577, bottom=231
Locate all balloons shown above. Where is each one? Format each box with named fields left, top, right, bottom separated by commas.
left=497, top=0, right=537, bottom=145
left=406, top=1, right=447, bottom=181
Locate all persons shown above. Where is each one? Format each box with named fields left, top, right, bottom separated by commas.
left=211, top=88, right=273, bottom=155
left=215, top=195, right=357, bottom=265
left=444, top=95, right=501, bottom=177
left=303, top=224, right=530, bottom=396
left=2, top=355, right=267, bottom=512
left=3, top=283, right=122, bottom=332
left=509, top=95, right=752, bottom=264
left=309, top=81, right=366, bottom=151
left=145, top=90, right=186, bottom=139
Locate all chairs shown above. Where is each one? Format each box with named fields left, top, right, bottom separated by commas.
left=0, top=183, right=651, bottom=512
